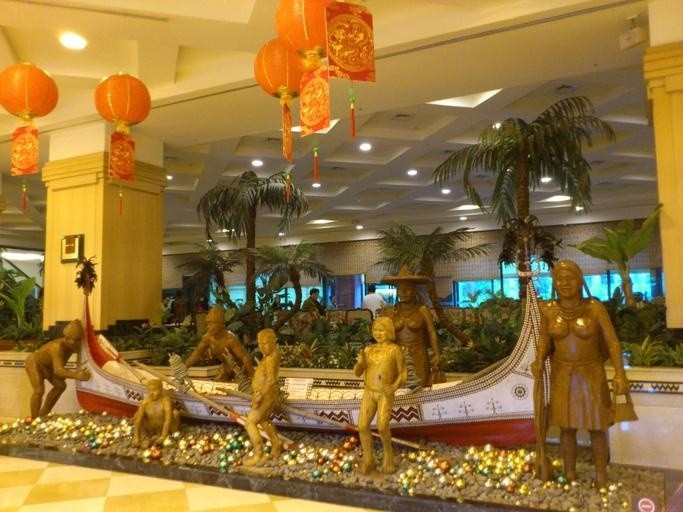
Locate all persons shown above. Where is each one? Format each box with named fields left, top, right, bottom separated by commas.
left=351, top=316, right=408, bottom=475
left=156, top=288, right=225, bottom=326
left=301, top=289, right=328, bottom=321
left=126, top=378, right=182, bottom=448
left=326, top=295, right=336, bottom=311
left=359, top=285, right=385, bottom=319
left=270, top=297, right=282, bottom=311
left=23, top=318, right=90, bottom=417
left=183, top=302, right=256, bottom=381
left=527, top=260, right=630, bottom=484
left=238, top=327, right=282, bottom=466
left=377, top=263, right=440, bottom=390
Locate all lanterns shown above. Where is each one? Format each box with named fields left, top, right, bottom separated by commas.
left=272, top=1, right=360, bottom=182
left=0, top=60, right=59, bottom=211
left=93, top=71, right=152, bottom=217
left=253, top=34, right=313, bottom=204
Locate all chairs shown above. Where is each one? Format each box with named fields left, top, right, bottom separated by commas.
left=377, top=307, right=396, bottom=320
left=226, top=304, right=373, bottom=345
left=430, top=306, right=492, bottom=345
left=194, top=311, right=209, bottom=340
